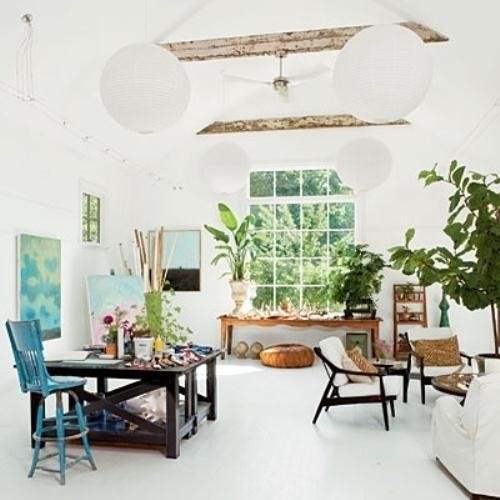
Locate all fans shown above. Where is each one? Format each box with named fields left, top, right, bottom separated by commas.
left=221, top=45, right=329, bottom=106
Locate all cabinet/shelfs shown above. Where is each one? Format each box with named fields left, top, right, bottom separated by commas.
left=390, top=282, right=430, bottom=364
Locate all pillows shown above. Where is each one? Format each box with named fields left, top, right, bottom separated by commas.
left=341, top=344, right=377, bottom=383
left=410, top=333, right=463, bottom=368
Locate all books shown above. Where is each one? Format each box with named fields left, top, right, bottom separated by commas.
left=43, top=351, right=95, bottom=362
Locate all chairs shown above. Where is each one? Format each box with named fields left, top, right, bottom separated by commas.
left=426, top=371, right=500, bottom=500
left=400, top=325, right=474, bottom=407
left=3, top=318, right=99, bottom=486
left=310, top=335, right=404, bottom=433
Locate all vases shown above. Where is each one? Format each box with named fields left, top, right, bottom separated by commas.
left=105, top=343, right=118, bottom=359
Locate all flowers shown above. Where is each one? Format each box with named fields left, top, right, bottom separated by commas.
left=99, top=314, right=117, bottom=344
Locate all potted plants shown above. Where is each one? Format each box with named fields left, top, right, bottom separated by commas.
left=394, top=282, right=416, bottom=321
left=198, top=199, right=271, bottom=314
left=385, top=157, right=500, bottom=374
left=326, top=238, right=386, bottom=312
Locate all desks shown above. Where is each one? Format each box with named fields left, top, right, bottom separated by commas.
left=215, top=312, right=386, bottom=362
left=426, top=373, right=474, bottom=401
left=12, top=346, right=225, bottom=460
